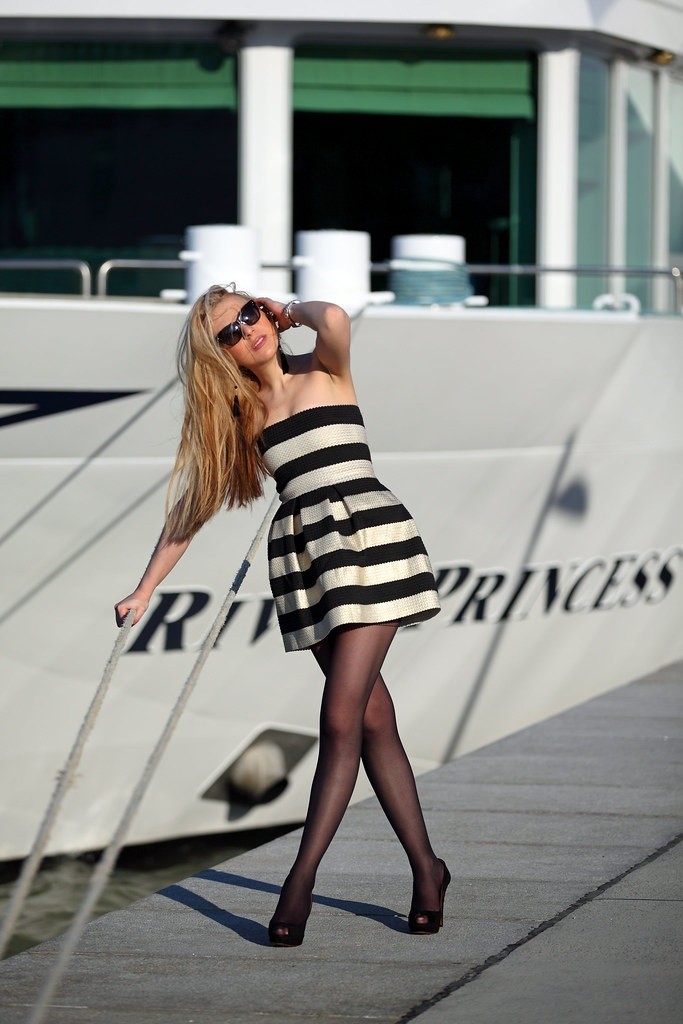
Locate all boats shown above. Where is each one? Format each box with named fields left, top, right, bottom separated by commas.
left=0, top=4, right=683, bottom=885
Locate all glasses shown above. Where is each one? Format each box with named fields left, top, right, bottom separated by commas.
left=215, top=299, right=260, bottom=347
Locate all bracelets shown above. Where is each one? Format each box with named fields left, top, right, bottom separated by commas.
left=283, top=299, right=304, bottom=329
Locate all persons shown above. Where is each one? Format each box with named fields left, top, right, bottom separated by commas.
left=113, top=284, right=452, bottom=948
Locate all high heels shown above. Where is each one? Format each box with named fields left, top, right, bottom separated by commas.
left=266, top=893, right=312, bottom=946
left=408, top=857, right=451, bottom=934
